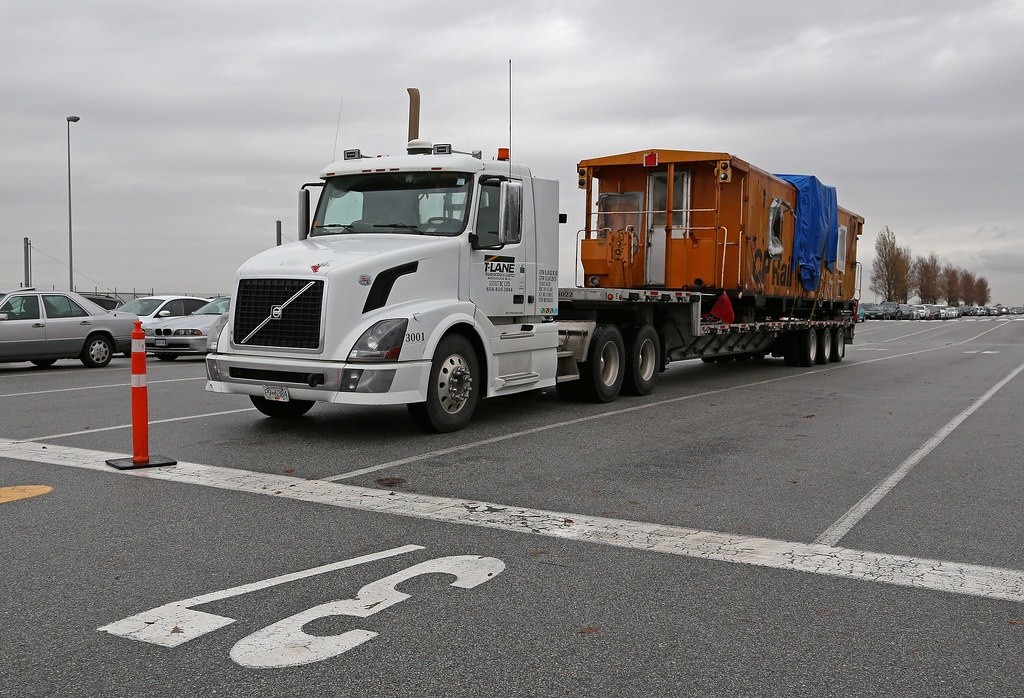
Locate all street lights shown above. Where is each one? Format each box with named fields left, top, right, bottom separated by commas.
left=67, top=116, right=79, bottom=292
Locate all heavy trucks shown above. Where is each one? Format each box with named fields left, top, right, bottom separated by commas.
left=205, top=57, right=845, bottom=434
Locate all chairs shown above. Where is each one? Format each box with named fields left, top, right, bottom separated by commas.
left=0, top=301, right=16, bottom=320
left=23, top=302, right=30, bottom=312
left=479, top=206, right=499, bottom=246
left=365, top=195, right=418, bottom=227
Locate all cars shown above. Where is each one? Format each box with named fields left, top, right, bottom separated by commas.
left=855, top=304, right=865, bottom=322
left=114, top=295, right=226, bottom=358
left=0, top=291, right=139, bottom=368
left=864, top=305, right=891, bottom=320
left=899, top=302, right=1024, bottom=320
left=144, top=296, right=231, bottom=361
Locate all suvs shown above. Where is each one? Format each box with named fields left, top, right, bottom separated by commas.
left=76, top=292, right=124, bottom=312
left=882, top=302, right=902, bottom=320
